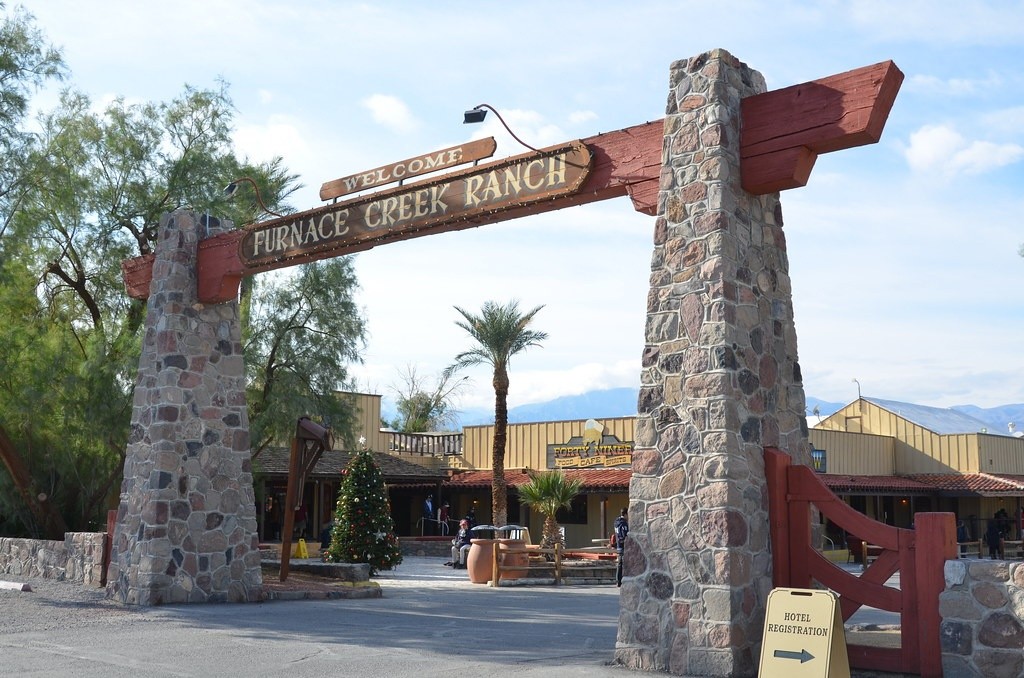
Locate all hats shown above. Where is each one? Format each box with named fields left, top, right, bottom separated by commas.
left=459, top=518, right=468, bottom=525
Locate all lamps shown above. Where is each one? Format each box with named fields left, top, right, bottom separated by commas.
left=222, top=177, right=282, bottom=218
left=999, top=497, right=1004, bottom=503
left=463, top=103, right=547, bottom=155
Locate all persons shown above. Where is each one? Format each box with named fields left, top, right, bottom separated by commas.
left=448, top=520, right=476, bottom=568
left=986, top=508, right=1024, bottom=560
left=465, top=507, right=479, bottom=538
left=613, top=508, right=629, bottom=587
left=424, top=494, right=450, bottom=536
left=293, top=499, right=308, bottom=538
left=957, top=520, right=969, bottom=558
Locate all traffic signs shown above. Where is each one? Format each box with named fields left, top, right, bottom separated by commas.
left=756, top=586, right=851, bottom=678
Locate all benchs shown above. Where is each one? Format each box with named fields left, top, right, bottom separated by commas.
left=846, top=535, right=882, bottom=563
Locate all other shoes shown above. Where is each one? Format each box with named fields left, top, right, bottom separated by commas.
left=444, top=562, right=452, bottom=567
left=453, top=563, right=464, bottom=569
left=617, top=583, right=622, bottom=588
